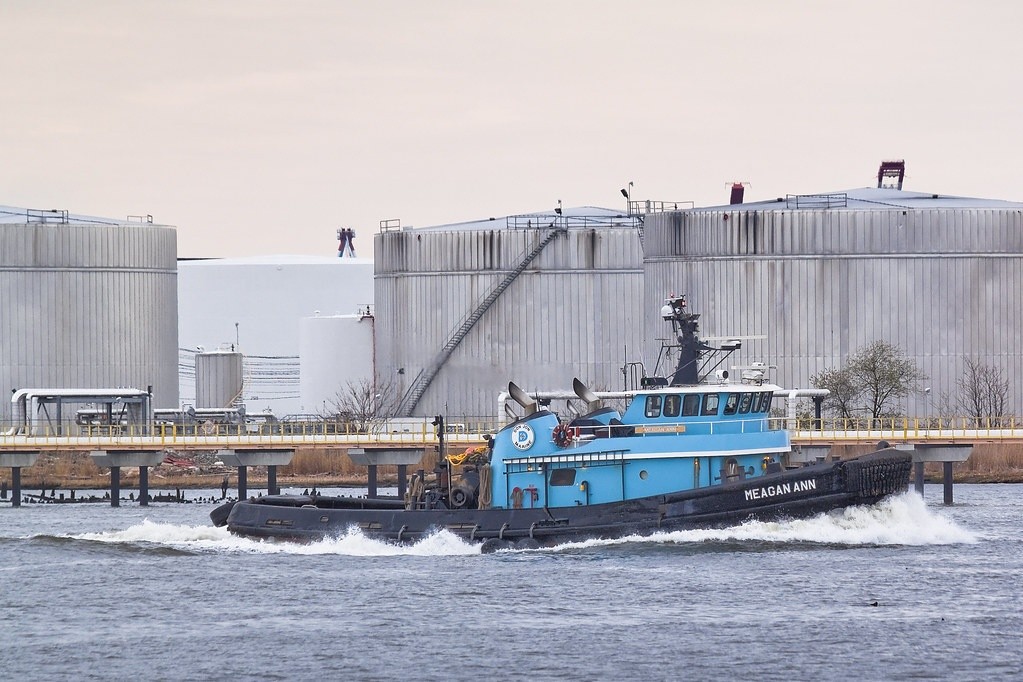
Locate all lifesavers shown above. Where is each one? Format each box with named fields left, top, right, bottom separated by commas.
left=552, top=425, right=572, bottom=447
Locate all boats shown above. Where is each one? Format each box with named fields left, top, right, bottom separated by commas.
left=227, top=294, right=911, bottom=550
